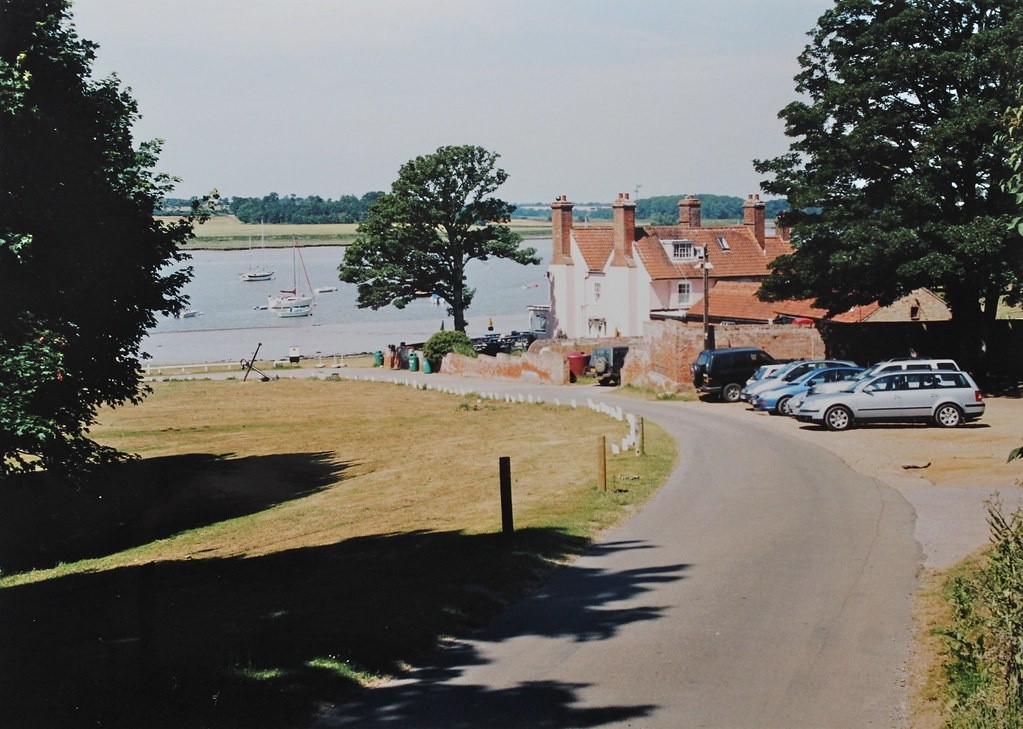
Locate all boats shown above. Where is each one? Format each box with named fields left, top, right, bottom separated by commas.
left=253, top=305, right=267, bottom=310
left=316, top=287, right=335, bottom=293
left=430, top=294, right=447, bottom=306
left=180, top=308, right=198, bottom=319
left=414, top=289, right=432, bottom=297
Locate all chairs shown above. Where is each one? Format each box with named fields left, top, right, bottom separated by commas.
left=895, top=377, right=942, bottom=389
left=824, top=375, right=829, bottom=383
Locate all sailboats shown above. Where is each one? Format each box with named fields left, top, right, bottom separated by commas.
left=270, top=232, right=317, bottom=318
left=238, top=219, right=275, bottom=283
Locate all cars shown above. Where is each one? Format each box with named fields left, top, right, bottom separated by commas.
left=743, top=356, right=964, bottom=418
left=794, top=370, right=985, bottom=433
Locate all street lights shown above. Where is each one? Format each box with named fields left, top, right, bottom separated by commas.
left=693, top=261, right=714, bottom=351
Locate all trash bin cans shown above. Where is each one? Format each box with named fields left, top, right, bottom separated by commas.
left=408, top=353, right=419, bottom=371
left=423, top=356, right=433, bottom=374
left=374, top=351, right=383, bottom=365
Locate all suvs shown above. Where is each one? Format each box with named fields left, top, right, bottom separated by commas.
left=586, top=347, right=628, bottom=386
left=689, top=346, right=806, bottom=402
